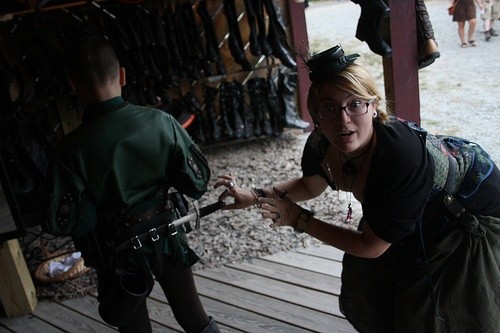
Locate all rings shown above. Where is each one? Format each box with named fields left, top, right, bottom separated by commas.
left=276, top=213, right=280, bottom=219
left=225, top=182, right=234, bottom=188
left=232, top=179, right=236, bottom=184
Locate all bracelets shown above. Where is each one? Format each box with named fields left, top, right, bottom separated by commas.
left=249, top=186, right=267, bottom=208
left=294, top=209, right=314, bottom=233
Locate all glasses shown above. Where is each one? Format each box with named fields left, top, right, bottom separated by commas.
left=316, top=99, right=370, bottom=119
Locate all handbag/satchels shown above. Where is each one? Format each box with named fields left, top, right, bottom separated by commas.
left=340, top=192, right=500, bottom=332
left=96, top=203, right=200, bottom=295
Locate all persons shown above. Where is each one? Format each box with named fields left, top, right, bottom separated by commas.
left=448, top=0, right=485, bottom=48
left=41, top=45, right=219, bottom=332
left=480, top=0, right=500, bottom=41
left=213, top=44, right=499, bottom=333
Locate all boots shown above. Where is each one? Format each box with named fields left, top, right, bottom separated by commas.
left=356, top=1, right=392, bottom=57
left=352, top=0, right=390, bottom=15
left=28, top=0, right=311, bottom=138
left=199, top=316, right=221, bottom=333
